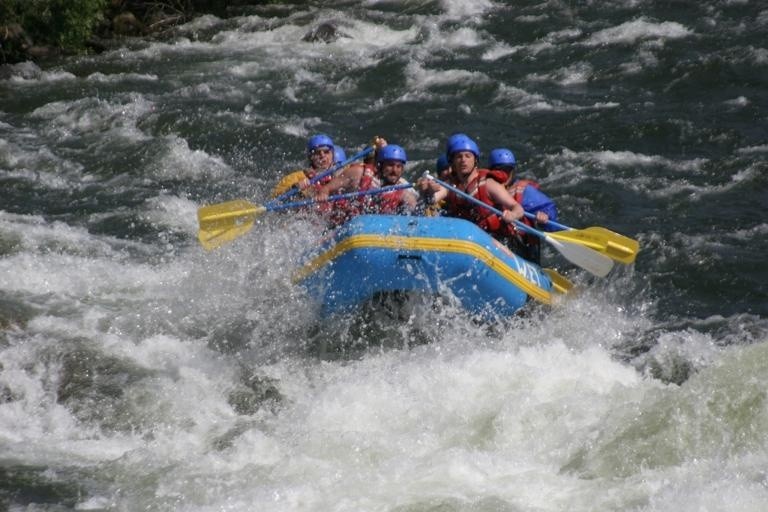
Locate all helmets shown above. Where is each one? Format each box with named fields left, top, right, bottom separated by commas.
left=307, top=135, right=347, bottom=165
left=375, top=143, right=407, bottom=164
left=488, top=148, right=516, bottom=170
left=436, top=155, right=450, bottom=177
left=447, top=134, right=480, bottom=162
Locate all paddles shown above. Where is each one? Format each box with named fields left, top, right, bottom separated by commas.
left=198, top=182, right=418, bottom=231
left=538, top=268, right=577, bottom=297
left=523, top=210, right=640, bottom=266
left=421, top=168, right=614, bottom=279
left=514, top=226, right=609, bottom=255
left=198, top=141, right=377, bottom=252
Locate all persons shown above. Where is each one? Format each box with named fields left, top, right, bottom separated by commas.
left=272, top=134, right=558, bottom=265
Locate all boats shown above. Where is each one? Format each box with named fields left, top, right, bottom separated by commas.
left=288, top=211, right=556, bottom=320
